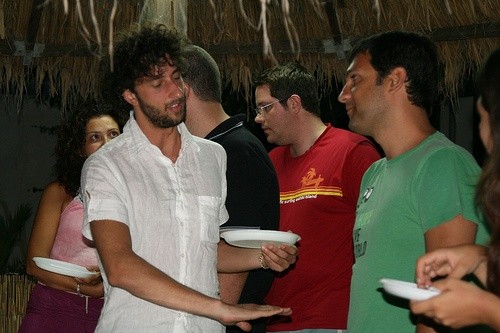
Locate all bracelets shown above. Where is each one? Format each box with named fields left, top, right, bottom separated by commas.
left=76, top=281, right=84, bottom=299
left=259, top=248, right=271, bottom=271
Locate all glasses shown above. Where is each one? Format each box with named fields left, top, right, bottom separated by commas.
left=255, top=95, right=291, bottom=116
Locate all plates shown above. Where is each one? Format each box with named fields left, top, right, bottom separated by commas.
left=220, top=230, right=301, bottom=248
left=378, top=278, right=441, bottom=300
left=32, top=257, right=97, bottom=279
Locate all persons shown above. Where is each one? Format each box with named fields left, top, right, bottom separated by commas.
left=82, top=19, right=298, bottom=333
left=338, top=31, right=492, bottom=333
left=18, top=105, right=122, bottom=333
left=178, top=44, right=382, bottom=333
left=409, top=47, right=500, bottom=333
